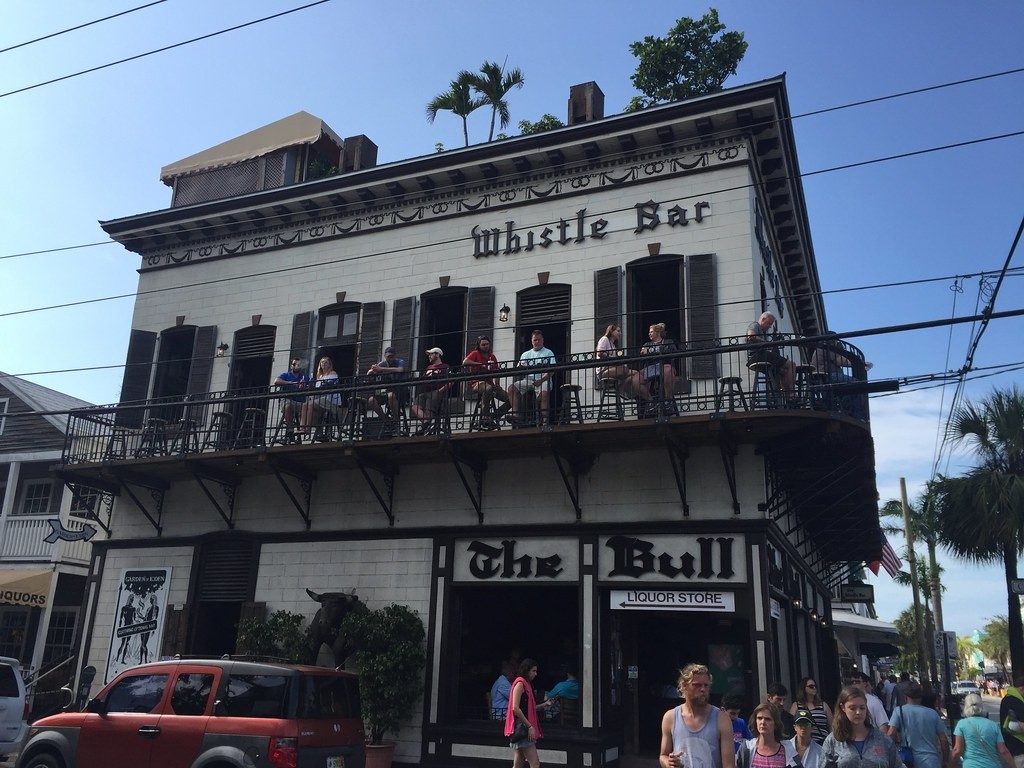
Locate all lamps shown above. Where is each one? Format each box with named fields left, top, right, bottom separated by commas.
left=809, top=607, right=819, bottom=622
left=500, top=304, right=510, bottom=321
left=216, top=341, right=228, bottom=357
left=790, top=594, right=803, bottom=611
left=819, top=616, right=827, bottom=627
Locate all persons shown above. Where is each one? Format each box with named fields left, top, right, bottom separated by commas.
left=747, top=312, right=798, bottom=398
left=596, top=324, right=637, bottom=399
left=735, top=673, right=1024, bottom=768
left=721, top=695, right=752, bottom=755
left=492, top=647, right=579, bottom=768
left=506, top=330, right=556, bottom=425
left=639, top=322, right=677, bottom=408
left=811, top=331, right=873, bottom=413
left=462, top=336, right=511, bottom=429
left=367, top=347, right=406, bottom=435
left=274, top=357, right=310, bottom=445
left=411, top=347, right=454, bottom=435
left=299, top=357, right=342, bottom=433
left=659, top=664, right=735, bottom=768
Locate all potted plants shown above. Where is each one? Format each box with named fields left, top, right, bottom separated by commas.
left=341, top=602, right=426, bottom=768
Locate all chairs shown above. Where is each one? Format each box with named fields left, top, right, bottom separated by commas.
left=486, top=692, right=493, bottom=720
left=559, top=698, right=580, bottom=730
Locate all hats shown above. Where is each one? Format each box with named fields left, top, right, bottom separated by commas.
left=426, top=347, right=443, bottom=356
left=793, top=710, right=813, bottom=723
left=385, top=347, right=396, bottom=356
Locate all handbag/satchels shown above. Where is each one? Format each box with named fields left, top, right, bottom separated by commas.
left=508, top=720, right=527, bottom=743
left=898, top=746, right=914, bottom=764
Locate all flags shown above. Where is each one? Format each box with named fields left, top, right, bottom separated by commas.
left=867, top=533, right=903, bottom=578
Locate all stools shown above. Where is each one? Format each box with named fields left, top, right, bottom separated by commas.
left=520, top=390, right=539, bottom=428
left=796, top=364, right=829, bottom=411
left=468, top=394, right=501, bottom=431
left=597, top=378, right=624, bottom=422
left=200, top=411, right=232, bottom=452
left=337, top=397, right=367, bottom=440
left=558, top=383, right=584, bottom=425
left=309, top=409, right=339, bottom=442
left=427, top=411, right=451, bottom=435
left=105, top=428, right=128, bottom=459
left=716, top=376, right=748, bottom=412
left=377, top=402, right=409, bottom=440
left=642, top=375, right=679, bottom=419
left=271, top=411, right=301, bottom=446
left=748, top=361, right=782, bottom=410
left=136, top=418, right=198, bottom=458
left=233, top=408, right=267, bottom=449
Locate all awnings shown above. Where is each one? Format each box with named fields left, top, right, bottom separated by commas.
left=0, top=569, right=53, bottom=609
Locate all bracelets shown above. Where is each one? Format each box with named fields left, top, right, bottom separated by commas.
left=527, top=725, right=532, bottom=730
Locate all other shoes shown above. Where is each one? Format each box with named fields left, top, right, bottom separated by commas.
left=481, top=417, right=496, bottom=428
left=316, top=434, right=329, bottom=442
left=292, top=429, right=308, bottom=434
left=505, top=415, right=520, bottom=423
left=288, top=431, right=295, bottom=442
left=648, top=402, right=660, bottom=411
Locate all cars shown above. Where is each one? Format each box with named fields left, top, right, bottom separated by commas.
left=953, top=681, right=982, bottom=697
left=0, top=658, right=31, bottom=761
left=11, top=653, right=367, bottom=768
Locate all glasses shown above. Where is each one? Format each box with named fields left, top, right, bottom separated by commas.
left=850, top=680, right=864, bottom=684
left=806, top=684, right=818, bottom=689
